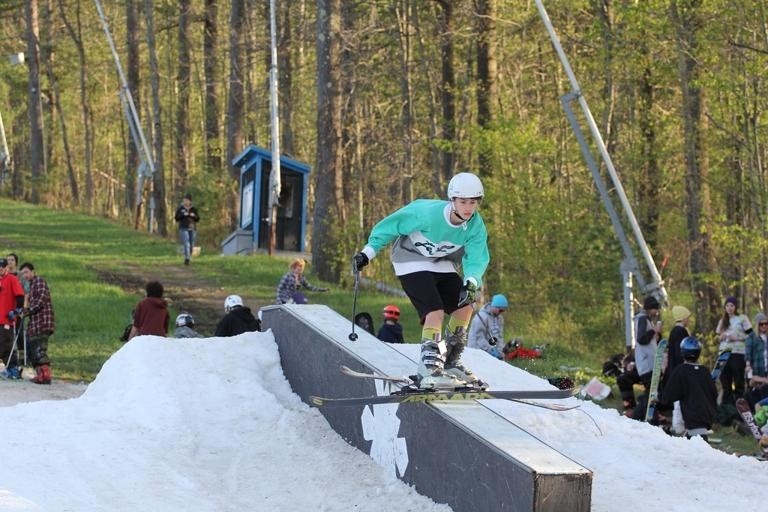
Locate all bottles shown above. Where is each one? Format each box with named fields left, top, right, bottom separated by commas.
left=656, top=321, right=663, bottom=326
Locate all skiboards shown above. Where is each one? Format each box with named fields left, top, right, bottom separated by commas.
left=645, top=339, right=732, bottom=422
left=306, top=365, right=581, bottom=412
left=736, top=398, right=767, bottom=460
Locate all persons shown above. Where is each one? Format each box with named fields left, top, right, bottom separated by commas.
left=18, top=262, right=55, bottom=384
left=175, top=194, right=200, bottom=264
left=276, top=259, right=331, bottom=304
left=354, top=173, right=490, bottom=389
left=119, top=309, right=135, bottom=341
left=215, top=295, right=261, bottom=337
left=467, top=294, right=508, bottom=360
left=128, top=281, right=170, bottom=342
left=0, top=259, right=25, bottom=379
left=174, top=314, right=206, bottom=338
left=6, top=254, right=31, bottom=365
left=376, top=305, right=405, bottom=343
left=617, top=296, right=768, bottom=462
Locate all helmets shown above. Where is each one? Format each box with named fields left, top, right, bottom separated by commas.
left=383, top=305, right=400, bottom=320
left=447, top=171, right=486, bottom=202
left=679, top=336, right=701, bottom=360
left=223, top=294, right=244, bottom=315
left=175, top=313, right=195, bottom=327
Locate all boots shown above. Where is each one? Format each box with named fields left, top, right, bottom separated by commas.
left=447, top=325, right=483, bottom=386
left=30, top=365, right=52, bottom=385
left=417, top=341, right=462, bottom=387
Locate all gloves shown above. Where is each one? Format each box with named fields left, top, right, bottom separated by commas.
left=457, top=280, right=476, bottom=308
left=350, top=252, right=369, bottom=272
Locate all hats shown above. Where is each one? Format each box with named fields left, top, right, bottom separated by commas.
left=725, top=296, right=738, bottom=307
left=671, top=305, right=693, bottom=322
left=643, top=296, right=660, bottom=310
left=491, top=294, right=508, bottom=308
